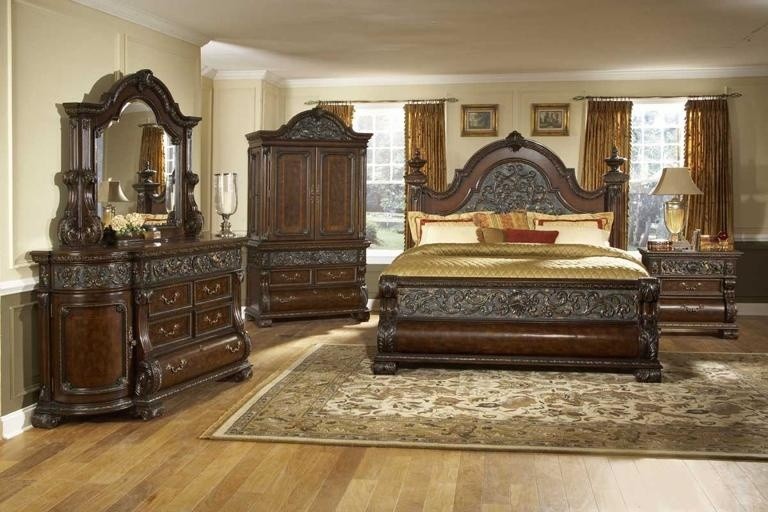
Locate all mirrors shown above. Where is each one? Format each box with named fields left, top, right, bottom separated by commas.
left=58, top=66, right=203, bottom=245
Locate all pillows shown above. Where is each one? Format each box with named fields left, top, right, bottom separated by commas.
left=406, top=211, right=614, bottom=245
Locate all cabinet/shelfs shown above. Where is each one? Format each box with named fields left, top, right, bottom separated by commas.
left=28, top=236, right=253, bottom=430
left=244, top=107, right=373, bottom=328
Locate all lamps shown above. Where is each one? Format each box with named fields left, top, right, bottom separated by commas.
left=648, top=168, right=704, bottom=249
left=212, top=171, right=239, bottom=237
left=96, top=177, right=130, bottom=225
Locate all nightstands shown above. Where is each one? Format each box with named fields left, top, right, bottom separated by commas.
left=637, top=246, right=744, bottom=340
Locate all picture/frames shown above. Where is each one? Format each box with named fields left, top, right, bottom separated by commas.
left=530, top=103, right=570, bottom=136
left=460, top=104, right=498, bottom=136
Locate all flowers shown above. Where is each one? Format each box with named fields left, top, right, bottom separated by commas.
left=101, top=212, right=149, bottom=245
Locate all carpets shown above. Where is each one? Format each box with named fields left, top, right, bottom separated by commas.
left=195, top=342, right=768, bottom=461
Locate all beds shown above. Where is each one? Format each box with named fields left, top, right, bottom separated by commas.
left=373, top=129, right=663, bottom=383
